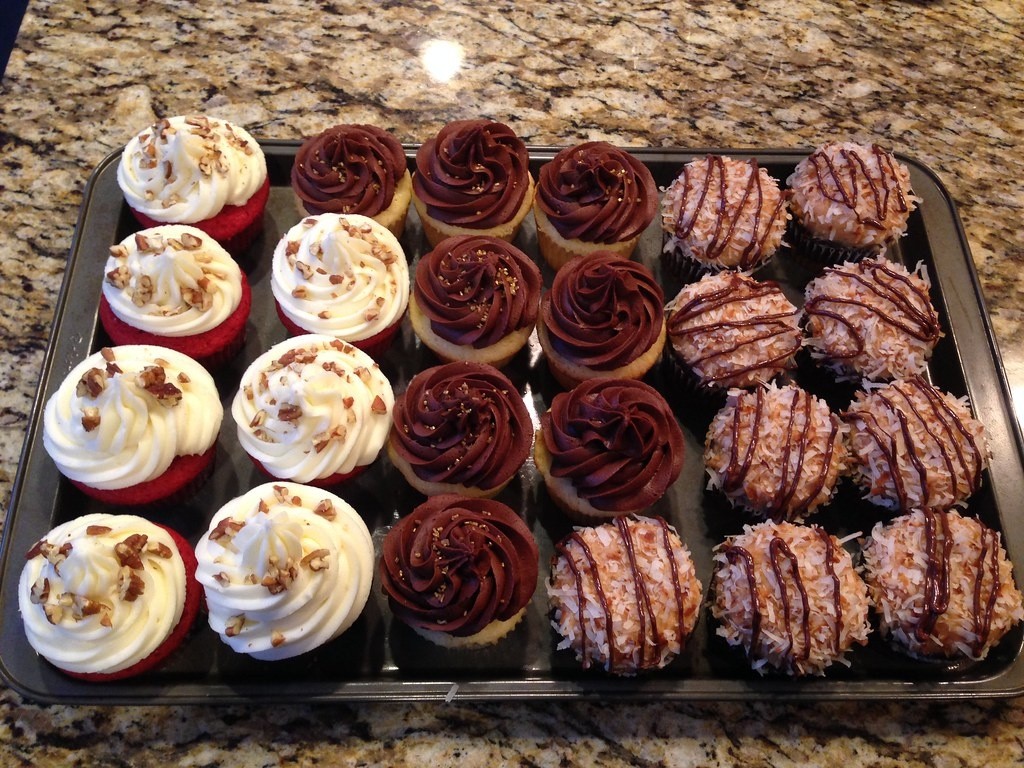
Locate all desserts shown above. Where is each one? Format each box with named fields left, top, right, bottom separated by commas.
left=18, top=115, right=1024, bottom=682
